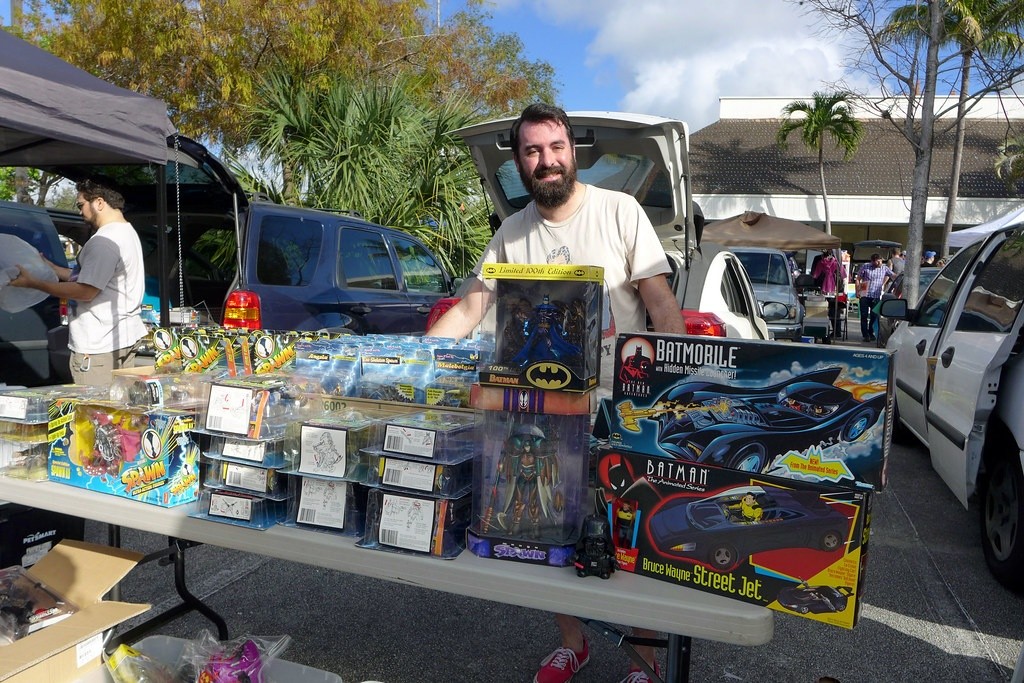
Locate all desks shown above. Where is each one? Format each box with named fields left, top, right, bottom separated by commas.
left=0, top=476, right=775, bottom=683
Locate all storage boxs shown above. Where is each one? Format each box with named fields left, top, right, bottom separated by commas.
left=152, top=322, right=351, bottom=374
left=0, top=537, right=153, bottom=683
left=362, top=453, right=472, bottom=499
left=276, top=407, right=403, bottom=484
left=463, top=382, right=598, bottom=567
left=188, top=371, right=329, bottom=443
left=202, top=437, right=290, bottom=470
left=359, top=409, right=482, bottom=466
left=0, top=385, right=106, bottom=481
left=78, top=633, right=343, bottom=683
left=202, top=460, right=292, bottom=501
left=595, top=444, right=873, bottom=630
left=274, top=475, right=365, bottom=539
left=353, top=488, right=472, bottom=560
left=48, top=398, right=200, bottom=509
left=186, top=488, right=285, bottom=531
left=612, top=332, right=897, bottom=493
left=479, top=262, right=605, bottom=393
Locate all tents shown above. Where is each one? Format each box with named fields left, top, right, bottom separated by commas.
left=0, top=17, right=165, bottom=331
left=701, top=209, right=842, bottom=341
left=947, top=204, right=1024, bottom=249
left=848, top=238, right=902, bottom=251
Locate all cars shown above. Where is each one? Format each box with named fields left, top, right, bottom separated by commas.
left=875, top=265, right=946, bottom=350
left=0, top=200, right=75, bottom=388
left=721, top=244, right=816, bottom=344
left=451, top=108, right=775, bottom=359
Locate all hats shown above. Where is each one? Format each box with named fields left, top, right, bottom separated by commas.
left=924, top=251, right=936, bottom=259
left=891, top=248, right=900, bottom=255
left=901, top=251, right=906, bottom=255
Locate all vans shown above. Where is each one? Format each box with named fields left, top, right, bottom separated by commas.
left=880, top=202, right=1024, bottom=595
left=75, top=133, right=471, bottom=340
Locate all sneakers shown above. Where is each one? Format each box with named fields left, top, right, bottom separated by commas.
left=618, top=660, right=660, bottom=683
left=533, top=635, right=589, bottom=683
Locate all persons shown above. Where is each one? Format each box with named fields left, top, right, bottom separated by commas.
left=854, top=246, right=936, bottom=342
left=415, top=98, right=687, bottom=683
left=488, top=425, right=558, bottom=540
left=830, top=277, right=845, bottom=336
left=9, top=172, right=151, bottom=404
left=510, top=292, right=581, bottom=369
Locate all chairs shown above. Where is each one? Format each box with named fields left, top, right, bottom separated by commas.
left=827, top=293, right=848, bottom=342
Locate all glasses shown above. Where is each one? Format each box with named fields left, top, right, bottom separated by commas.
left=76, top=199, right=96, bottom=210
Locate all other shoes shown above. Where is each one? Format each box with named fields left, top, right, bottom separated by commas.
left=869, top=333, right=876, bottom=340
left=830, top=332, right=841, bottom=337
left=864, top=334, right=870, bottom=341
